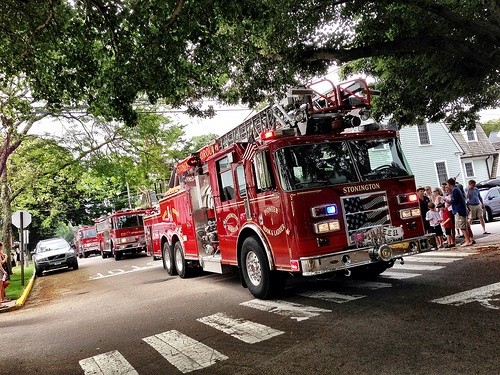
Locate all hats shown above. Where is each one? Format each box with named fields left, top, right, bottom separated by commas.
left=436, top=203, right=445, bottom=208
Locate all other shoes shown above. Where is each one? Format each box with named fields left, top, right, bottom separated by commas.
left=430, top=232, right=491, bottom=250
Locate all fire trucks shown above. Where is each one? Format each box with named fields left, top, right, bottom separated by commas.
left=144, top=79, right=425, bottom=298
left=95, top=207, right=157, bottom=261
left=73, top=226, right=97, bottom=258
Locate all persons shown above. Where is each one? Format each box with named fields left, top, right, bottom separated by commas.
left=0, top=242, right=10, bottom=303
left=416, top=177, right=491, bottom=250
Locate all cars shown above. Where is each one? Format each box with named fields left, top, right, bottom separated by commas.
left=31, top=239, right=79, bottom=276
left=466, top=177, right=500, bottom=221
